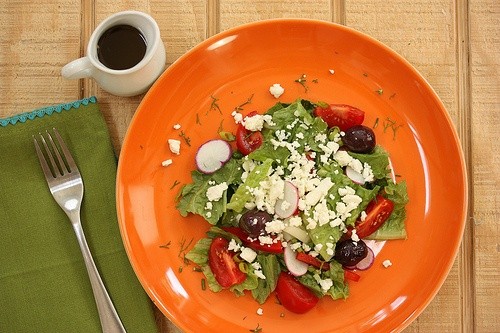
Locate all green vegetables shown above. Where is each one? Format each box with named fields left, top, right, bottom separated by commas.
left=175, top=99, right=409, bottom=304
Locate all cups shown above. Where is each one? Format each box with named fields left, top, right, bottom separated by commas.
left=60, top=10, right=167, bottom=99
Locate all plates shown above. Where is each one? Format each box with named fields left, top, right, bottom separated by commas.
left=115, top=18, right=469, bottom=333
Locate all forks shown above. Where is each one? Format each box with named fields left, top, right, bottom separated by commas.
left=32, top=128, right=128, bottom=333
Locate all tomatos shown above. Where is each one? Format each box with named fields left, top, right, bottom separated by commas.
left=275, top=271, right=319, bottom=313
